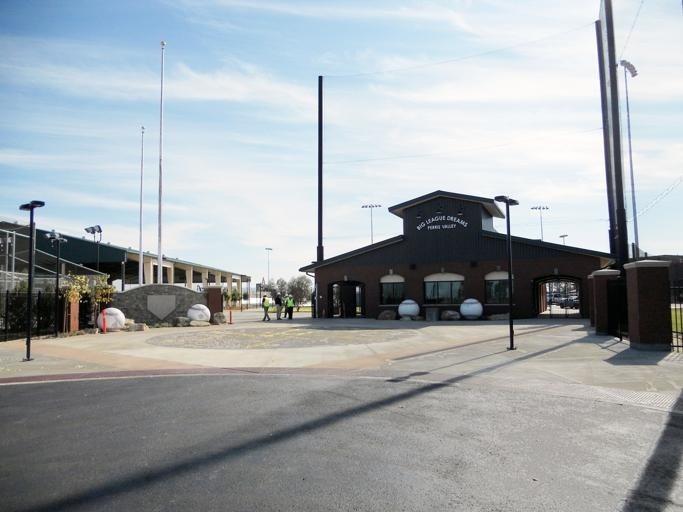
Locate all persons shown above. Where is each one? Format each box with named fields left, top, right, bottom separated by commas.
left=281, top=293, right=288, bottom=318
left=261, top=293, right=271, bottom=321
left=285, top=294, right=296, bottom=318
left=275, top=292, right=283, bottom=320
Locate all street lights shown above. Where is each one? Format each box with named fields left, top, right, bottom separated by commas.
left=620, top=60, right=639, bottom=244
left=19, top=200, right=102, bottom=362
left=361, top=204, right=382, bottom=244
left=265, top=248, right=272, bottom=285
left=531, top=207, right=568, bottom=246
left=494, top=195, right=519, bottom=351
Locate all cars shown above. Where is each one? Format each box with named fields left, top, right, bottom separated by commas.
left=546, top=289, right=580, bottom=309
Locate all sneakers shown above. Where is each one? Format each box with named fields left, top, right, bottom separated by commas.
left=261, top=316, right=293, bottom=321
